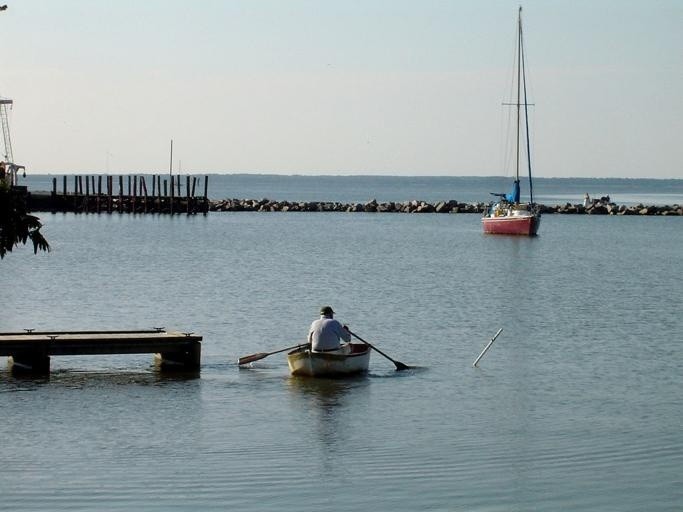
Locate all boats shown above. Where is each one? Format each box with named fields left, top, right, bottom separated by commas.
left=287, top=341, right=370, bottom=375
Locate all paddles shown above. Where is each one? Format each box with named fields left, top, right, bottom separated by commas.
left=348, top=331, right=410, bottom=371
left=239, top=343, right=309, bottom=365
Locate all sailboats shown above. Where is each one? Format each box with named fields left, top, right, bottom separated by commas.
left=479, top=6, right=543, bottom=237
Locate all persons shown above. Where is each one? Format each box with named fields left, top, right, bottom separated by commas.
left=584, top=192, right=590, bottom=206
left=308, top=306, right=352, bottom=354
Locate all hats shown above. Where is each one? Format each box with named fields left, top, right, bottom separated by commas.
left=320, top=306, right=336, bottom=315
